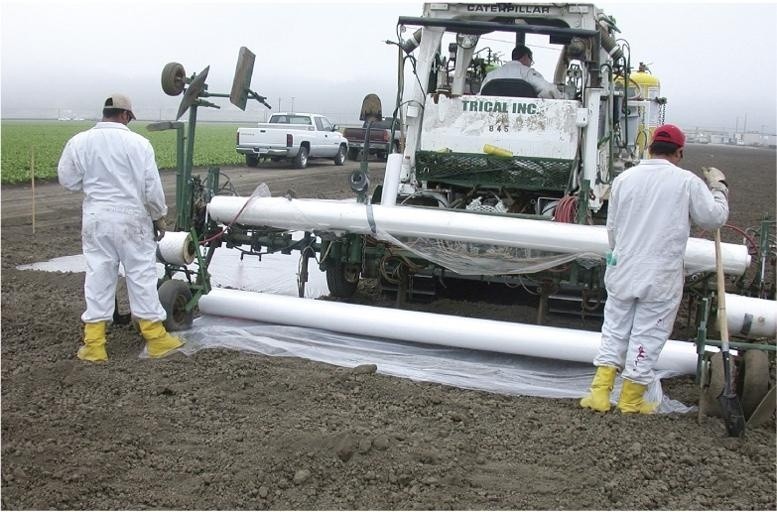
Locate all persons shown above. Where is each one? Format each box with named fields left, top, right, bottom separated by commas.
left=476, top=45, right=567, bottom=99
left=578, top=124, right=730, bottom=414
left=56, top=91, right=186, bottom=365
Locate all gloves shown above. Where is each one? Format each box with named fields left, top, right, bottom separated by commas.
left=153, top=217, right=167, bottom=242
left=701, top=166, right=729, bottom=199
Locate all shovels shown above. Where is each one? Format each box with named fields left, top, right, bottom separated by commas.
left=713, top=227, right=747, bottom=438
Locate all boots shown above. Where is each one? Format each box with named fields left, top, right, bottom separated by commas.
left=77, top=320, right=109, bottom=362
left=580, top=366, right=617, bottom=411
left=616, top=379, right=659, bottom=415
left=138, top=319, right=183, bottom=357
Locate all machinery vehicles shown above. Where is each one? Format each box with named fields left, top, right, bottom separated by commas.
left=119, top=1, right=774, bottom=431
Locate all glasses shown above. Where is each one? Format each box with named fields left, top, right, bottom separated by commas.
left=529, top=55, right=534, bottom=65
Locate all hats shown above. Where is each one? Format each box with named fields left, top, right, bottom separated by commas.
left=653, top=124, right=686, bottom=160
left=102, top=94, right=137, bottom=120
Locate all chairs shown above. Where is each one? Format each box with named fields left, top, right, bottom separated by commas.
left=480, top=79, right=539, bottom=98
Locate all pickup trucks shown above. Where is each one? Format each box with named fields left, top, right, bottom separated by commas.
left=233, top=112, right=348, bottom=169
left=342, top=115, right=400, bottom=161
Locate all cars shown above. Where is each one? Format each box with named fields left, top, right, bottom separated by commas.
left=686, top=136, right=746, bottom=147
